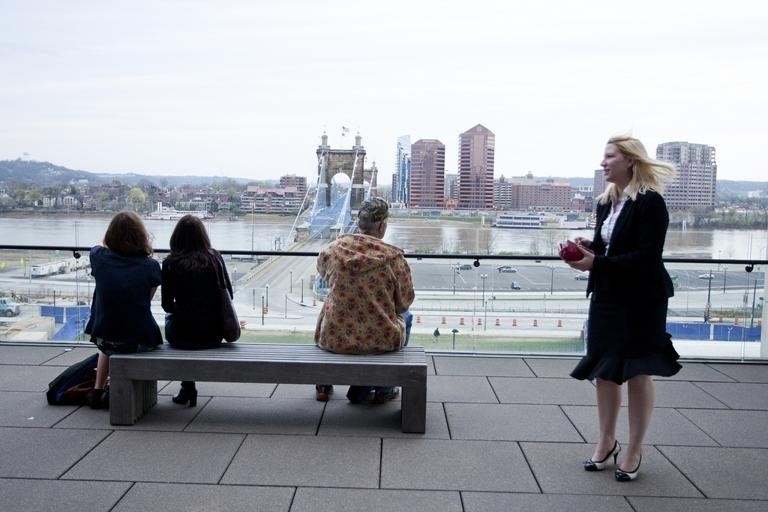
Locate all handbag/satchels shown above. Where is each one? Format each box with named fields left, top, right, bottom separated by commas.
left=220, top=288, right=240, bottom=343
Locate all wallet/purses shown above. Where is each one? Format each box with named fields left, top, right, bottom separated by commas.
left=558, top=240, right=595, bottom=261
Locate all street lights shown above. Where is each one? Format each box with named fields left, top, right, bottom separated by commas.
left=265, top=283, right=269, bottom=307
left=83, top=276, right=93, bottom=305
left=52, top=288, right=57, bottom=306
left=233, top=267, right=237, bottom=292
left=450, top=264, right=460, bottom=295
left=480, top=274, right=488, bottom=307
left=722, top=266, right=728, bottom=294
left=545, top=264, right=561, bottom=295
left=299, top=276, right=304, bottom=304
left=451, top=327, right=459, bottom=351
left=289, top=269, right=293, bottom=294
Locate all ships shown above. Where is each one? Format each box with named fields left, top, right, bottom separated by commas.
left=143, top=201, right=208, bottom=221
left=493, top=213, right=588, bottom=230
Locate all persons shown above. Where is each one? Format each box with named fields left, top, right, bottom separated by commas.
left=554, top=135, right=683, bottom=482
left=158, top=216, right=240, bottom=410
left=314, top=199, right=414, bottom=404
left=83, top=209, right=163, bottom=409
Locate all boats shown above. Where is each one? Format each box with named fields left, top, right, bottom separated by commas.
left=229, top=215, right=239, bottom=221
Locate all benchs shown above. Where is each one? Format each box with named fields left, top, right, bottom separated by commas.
left=103, top=337, right=431, bottom=436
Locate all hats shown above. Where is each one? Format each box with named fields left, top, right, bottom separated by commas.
left=357, top=197, right=391, bottom=222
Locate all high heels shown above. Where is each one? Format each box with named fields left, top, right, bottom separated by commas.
left=615, top=454, right=642, bottom=482
left=173, top=382, right=197, bottom=407
left=583, top=440, right=621, bottom=471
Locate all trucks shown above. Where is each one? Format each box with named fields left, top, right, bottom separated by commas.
left=0, top=297, right=20, bottom=317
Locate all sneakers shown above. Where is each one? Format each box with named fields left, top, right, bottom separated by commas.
left=315, top=385, right=333, bottom=401
left=90, top=388, right=105, bottom=409
left=374, top=387, right=399, bottom=404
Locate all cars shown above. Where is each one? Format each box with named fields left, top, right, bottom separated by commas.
left=574, top=273, right=589, bottom=281
left=497, top=264, right=511, bottom=270
left=503, top=268, right=517, bottom=273
left=698, top=273, right=715, bottom=280
left=669, top=273, right=678, bottom=281
left=510, top=281, right=521, bottom=290
left=455, top=264, right=472, bottom=271
